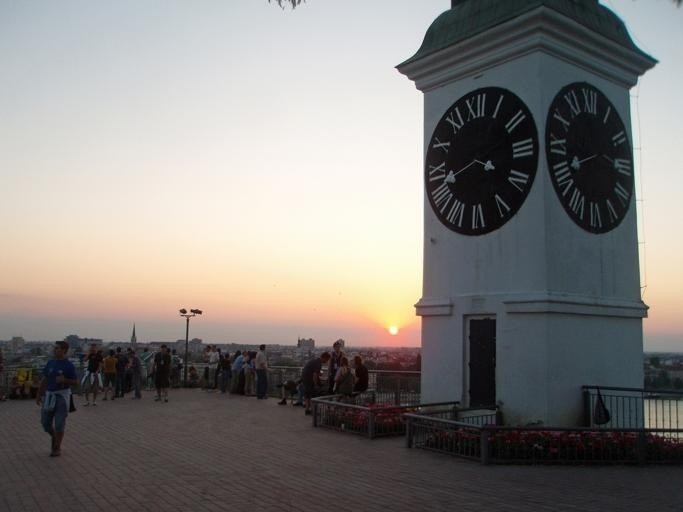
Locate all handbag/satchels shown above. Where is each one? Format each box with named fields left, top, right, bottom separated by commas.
left=67, top=393, right=77, bottom=412
left=332, top=381, right=337, bottom=392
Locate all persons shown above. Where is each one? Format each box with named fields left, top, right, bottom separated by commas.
left=34, top=340, right=79, bottom=457
left=101, top=338, right=370, bottom=417
left=78, top=343, right=100, bottom=406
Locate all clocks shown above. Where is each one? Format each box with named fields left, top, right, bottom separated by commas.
left=424, top=87, right=539, bottom=235
left=544, top=78, right=633, bottom=233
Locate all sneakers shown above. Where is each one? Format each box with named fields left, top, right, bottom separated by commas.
left=305, top=410, right=312, bottom=415
left=83, top=400, right=90, bottom=406
left=49, top=447, right=62, bottom=457
left=101, top=388, right=268, bottom=403
left=293, top=402, right=302, bottom=406
left=275, top=383, right=282, bottom=388
left=51, top=438, right=56, bottom=450
left=93, top=401, right=97, bottom=405
left=277, top=400, right=287, bottom=405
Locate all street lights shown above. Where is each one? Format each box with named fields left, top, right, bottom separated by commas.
left=179, top=308, right=202, bottom=388
